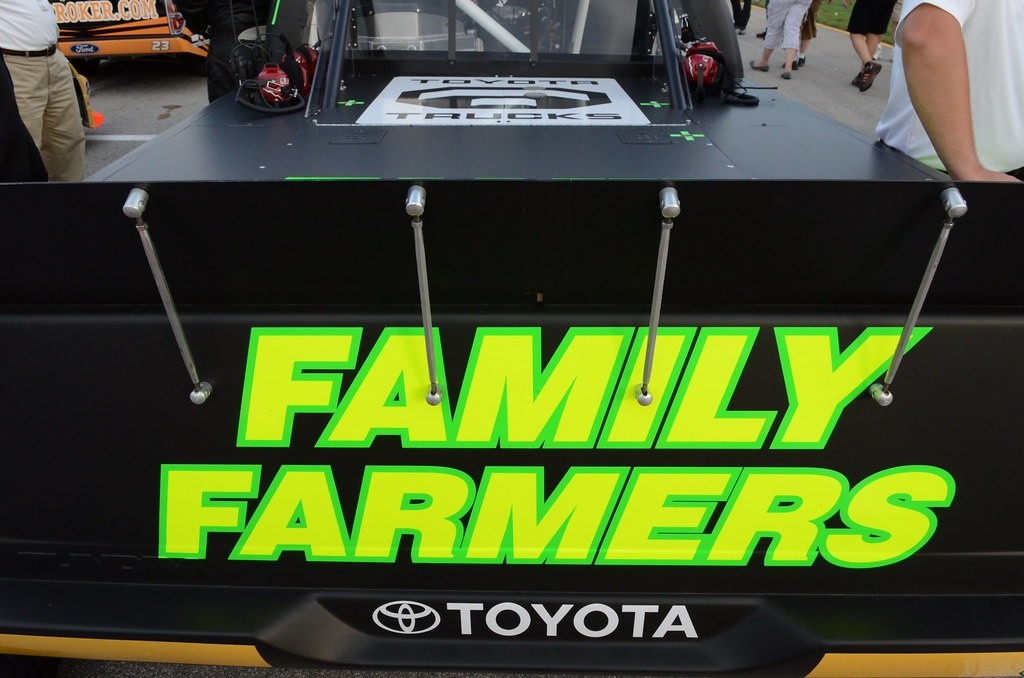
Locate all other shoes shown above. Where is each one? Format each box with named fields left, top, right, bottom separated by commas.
left=798, top=57, right=806, bottom=66
left=781, top=72, right=791, bottom=79
left=738, top=29, right=746, bottom=34
left=757, top=31, right=766, bottom=38
left=783, top=61, right=799, bottom=70
left=750, top=60, right=770, bottom=72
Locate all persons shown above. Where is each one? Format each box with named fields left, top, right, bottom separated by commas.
left=0, top=0, right=88, bottom=183
left=172, top=0, right=270, bottom=105
left=875, top=0, right=1024, bottom=182
left=731, top=0, right=898, bottom=92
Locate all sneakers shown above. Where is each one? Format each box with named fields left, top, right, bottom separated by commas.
left=852, top=61, right=882, bottom=91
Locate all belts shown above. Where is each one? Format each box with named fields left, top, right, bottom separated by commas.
left=0, top=44, right=56, bottom=57
